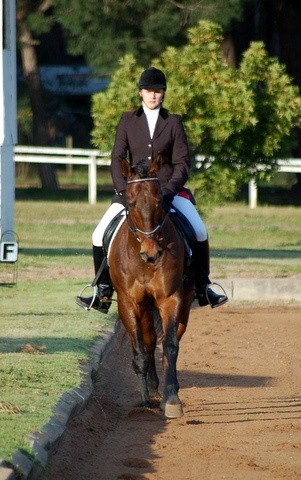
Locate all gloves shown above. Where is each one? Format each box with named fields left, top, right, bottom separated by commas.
left=160, top=194, right=174, bottom=213
left=112, top=190, right=127, bottom=206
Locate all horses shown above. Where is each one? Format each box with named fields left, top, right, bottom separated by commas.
left=102, top=155, right=196, bottom=419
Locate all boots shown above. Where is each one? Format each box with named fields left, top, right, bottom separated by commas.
left=75, top=245, right=114, bottom=314
left=193, top=239, right=228, bottom=308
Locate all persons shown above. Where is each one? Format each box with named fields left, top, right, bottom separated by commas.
left=73, top=66, right=228, bottom=314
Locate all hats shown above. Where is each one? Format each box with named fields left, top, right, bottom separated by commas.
left=139, top=67, right=166, bottom=89
